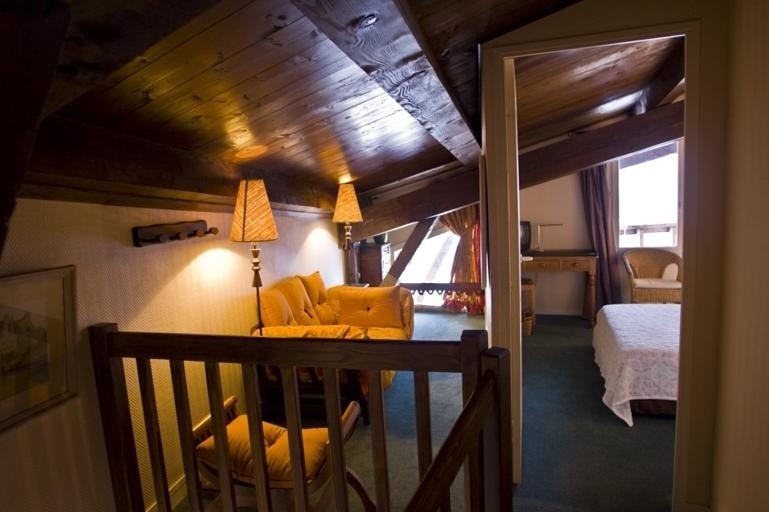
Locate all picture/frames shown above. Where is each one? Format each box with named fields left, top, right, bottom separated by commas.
left=0, top=264, right=80, bottom=431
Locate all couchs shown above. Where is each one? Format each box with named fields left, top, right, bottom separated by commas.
left=254, top=286, right=415, bottom=391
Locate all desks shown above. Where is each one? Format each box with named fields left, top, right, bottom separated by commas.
left=342, top=282, right=370, bottom=287
left=520, top=248, right=600, bottom=325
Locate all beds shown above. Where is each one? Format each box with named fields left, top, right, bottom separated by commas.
left=589, top=299, right=682, bottom=427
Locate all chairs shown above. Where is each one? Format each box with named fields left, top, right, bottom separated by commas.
left=620, top=246, right=683, bottom=303
left=191, top=395, right=383, bottom=511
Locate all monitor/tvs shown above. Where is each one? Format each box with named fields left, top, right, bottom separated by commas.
left=520, top=221, right=531, bottom=255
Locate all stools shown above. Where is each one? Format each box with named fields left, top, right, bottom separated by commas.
left=523, top=277, right=536, bottom=329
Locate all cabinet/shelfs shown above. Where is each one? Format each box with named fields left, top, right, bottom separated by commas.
left=359, top=242, right=393, bottom=284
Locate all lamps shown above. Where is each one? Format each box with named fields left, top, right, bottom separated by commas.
left=332, top=178, right=363, bottom=250
left=232, top=167, right=278, bottom=287
left=536, top=221, right=564, bottom=253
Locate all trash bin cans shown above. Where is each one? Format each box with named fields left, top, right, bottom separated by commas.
left=521, top=313, right=534, bottom=337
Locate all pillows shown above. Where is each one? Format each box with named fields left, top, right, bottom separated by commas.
left=258, top=270, right=405, bottom=327
left=195, top=412, right=328, bottom=484
